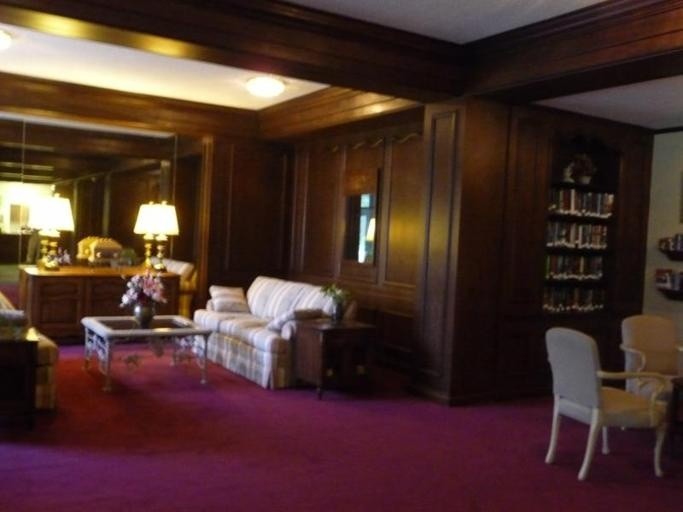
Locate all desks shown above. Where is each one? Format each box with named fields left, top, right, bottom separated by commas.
left=0, top=339, right=38, bottom=432
left=292, top=318, right=375, bottom=400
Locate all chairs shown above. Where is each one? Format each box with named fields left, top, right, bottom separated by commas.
left=619, top=314, right=682, bottom=432
left=544, top=327, right=670, bottom=482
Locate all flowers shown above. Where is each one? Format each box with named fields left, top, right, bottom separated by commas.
left=119, top=270, right=169, bottom=309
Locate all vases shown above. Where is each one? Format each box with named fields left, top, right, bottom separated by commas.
left=133, top=299, right=156, bottom=328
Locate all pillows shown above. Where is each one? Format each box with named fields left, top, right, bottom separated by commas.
left=208, top=285, right=251, bottom=313
left=265, top=309, right=322, bottom=332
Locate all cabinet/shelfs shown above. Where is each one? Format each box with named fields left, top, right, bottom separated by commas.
left=18, top=263, right=152, bottom=310
left=542, top=134, right=617, bottom=314
left=657, top=247, right=683, bottom=301
left=23, top=268, right=179, bottom=342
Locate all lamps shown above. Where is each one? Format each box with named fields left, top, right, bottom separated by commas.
left=150, top=200, right=180, bottom=271
left=27, top=194, right=49, bottom=267
left=133, top=201, right=156, bottom=269
left=42, top=193, right=75, bottom=271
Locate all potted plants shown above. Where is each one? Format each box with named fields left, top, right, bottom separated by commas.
left=320, top=281, right=352, bottom=326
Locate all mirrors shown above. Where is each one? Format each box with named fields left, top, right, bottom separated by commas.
left=0, top=110, right=178, bottom=333
left=343, top=191, right=376, bottom=265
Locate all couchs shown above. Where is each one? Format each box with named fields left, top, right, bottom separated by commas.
left=191, top=275, right=347, bottom=390
left=142, top=255, right=198, bottom=319
left=0, top=290, right=60, bottom=410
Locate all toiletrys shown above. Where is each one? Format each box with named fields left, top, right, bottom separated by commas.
left=542, top=186, right=615, bottom=313
